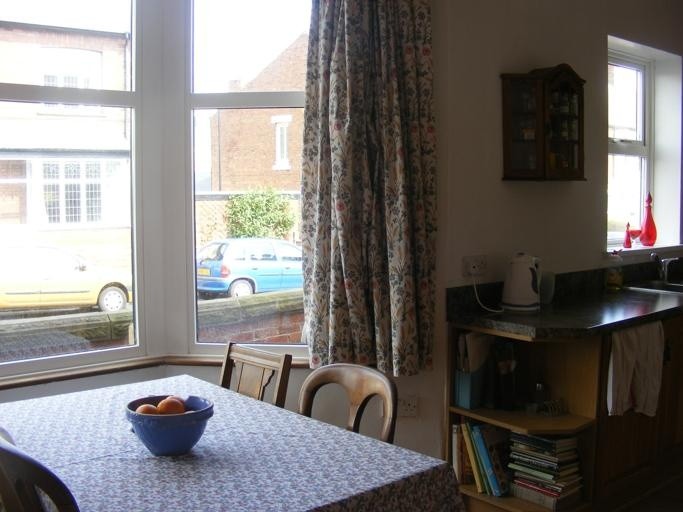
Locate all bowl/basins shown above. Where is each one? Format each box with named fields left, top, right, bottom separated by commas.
left=126, top=395, right=213, bottom=458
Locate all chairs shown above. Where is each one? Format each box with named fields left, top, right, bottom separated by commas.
left=0, top=429, right=82, bottom=511
left=216, top=340, right=292, bottom=408
left=296, top=365, right=397, bottom=443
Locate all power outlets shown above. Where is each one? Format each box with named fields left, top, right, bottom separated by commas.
left=464, top=256, right=487, bottom=277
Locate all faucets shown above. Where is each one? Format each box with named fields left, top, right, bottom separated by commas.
left=649, top=252, right=679, bottom=281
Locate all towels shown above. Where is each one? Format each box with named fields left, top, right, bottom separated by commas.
left=601, top=321, right=666, bottom=417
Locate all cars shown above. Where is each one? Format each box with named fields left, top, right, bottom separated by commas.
left=1, top=246, right=133, bottom=313
left=196, top=238, right=303, bottom=300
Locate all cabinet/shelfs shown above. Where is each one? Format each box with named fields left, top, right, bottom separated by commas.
left=502, top=64, right=587, bottom=184
left=443, top=283, right=682, bottom=512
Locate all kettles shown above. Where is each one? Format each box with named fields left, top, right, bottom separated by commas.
left=500, top=252, right=542, bottom=310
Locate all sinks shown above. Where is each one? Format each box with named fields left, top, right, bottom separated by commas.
left=621, top=279, right=683, bottom=295
